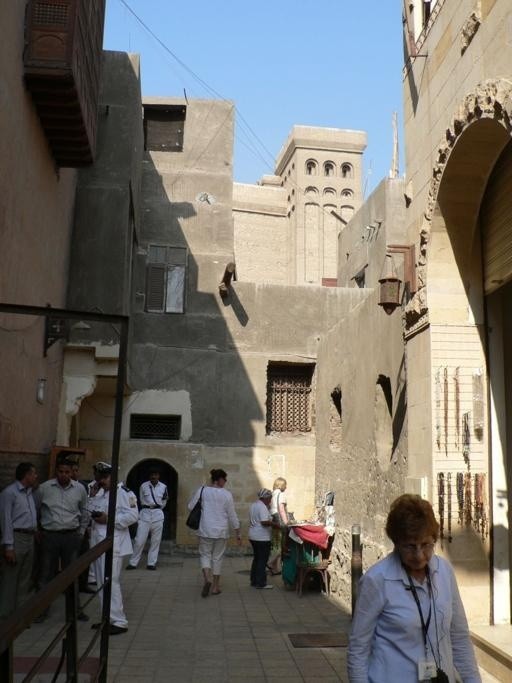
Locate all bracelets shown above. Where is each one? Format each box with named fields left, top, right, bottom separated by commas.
left=236, top=534, right=241, bottom=537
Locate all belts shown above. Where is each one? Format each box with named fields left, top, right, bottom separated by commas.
left=13, top=527, right=37, bottom=536
left=140, top=505, right=161, bottom=510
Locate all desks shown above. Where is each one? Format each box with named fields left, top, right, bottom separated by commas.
left=279, top=522, right=330, bottom=589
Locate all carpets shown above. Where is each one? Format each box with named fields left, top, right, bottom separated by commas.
left=288, top=630, right=350, bottom=648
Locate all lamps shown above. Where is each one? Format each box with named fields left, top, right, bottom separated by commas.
left=377, top=241, right=416, bottom=316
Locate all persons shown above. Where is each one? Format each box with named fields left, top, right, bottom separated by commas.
left=265, top=477, right=291, bottom=574
left=346, top=492, right=485, bottom=683
left=247, top=487, right=280, bottom=588
left=0, top=460, right=138, bottom=635
left=187, top=468, right=241, bottom=597
left=124, top=465, right=170, bottom=569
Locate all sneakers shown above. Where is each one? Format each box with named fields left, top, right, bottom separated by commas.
left=146, top=565, right=158, bottom=571
left=80, top=587, right=96, bottom=596
left=255, top=584, right=275, bottom=590
left=90, top=622, right=129, bottom=636
left=125, top=565, right=137, bottom=570
left=76, top=610, right=91, bottom=623
left=26, top=612, right=51, bottom=630
left=87, top=582, right=99, bottom=586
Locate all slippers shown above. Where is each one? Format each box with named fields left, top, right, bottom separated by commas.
left=200, top=580, right=213, bottom=598
left=211, top=587, right=224, bottom=595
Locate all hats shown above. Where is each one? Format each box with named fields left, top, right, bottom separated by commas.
left=94, top=460, right=122, bottom=474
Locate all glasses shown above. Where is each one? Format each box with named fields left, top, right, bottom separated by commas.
left=398, top=538, right=436, bottom=555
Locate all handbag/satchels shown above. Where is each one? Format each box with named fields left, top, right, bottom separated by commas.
left=185, top=485, right=205, bottom=531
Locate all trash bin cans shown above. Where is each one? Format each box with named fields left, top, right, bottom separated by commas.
left=282, top=529, right=321, bottom=591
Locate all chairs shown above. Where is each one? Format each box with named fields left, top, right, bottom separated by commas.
left=295, top=540, right=329, bottom=597
